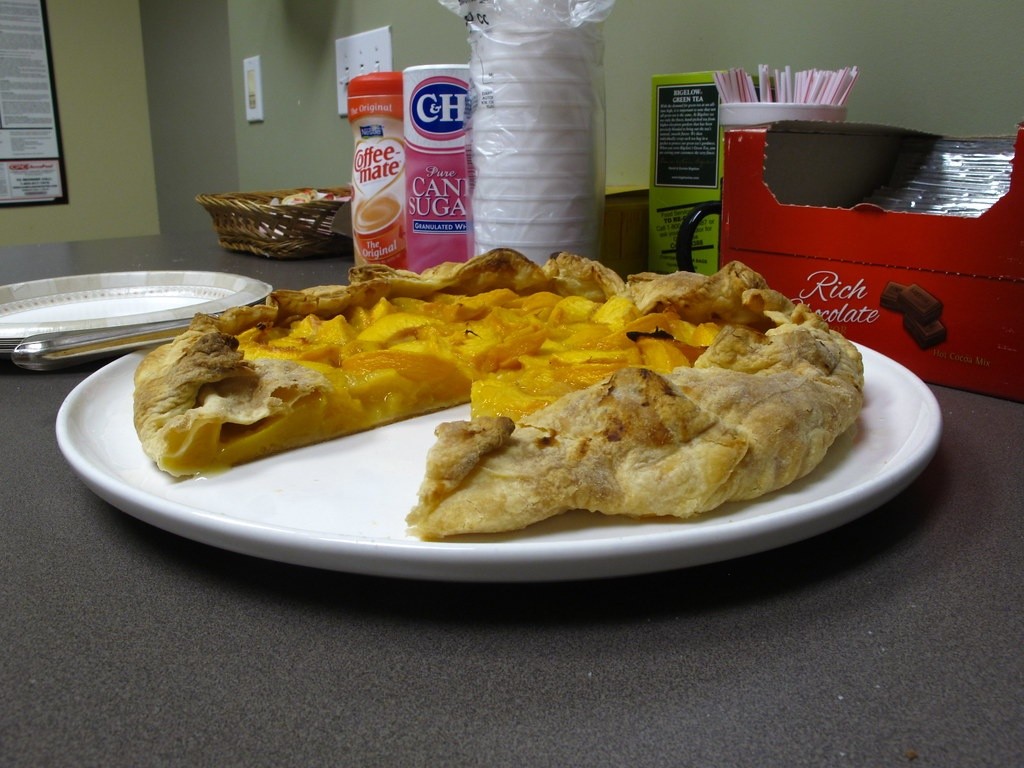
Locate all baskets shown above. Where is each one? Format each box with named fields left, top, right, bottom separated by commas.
left=195, top=186, right=357, bottom=259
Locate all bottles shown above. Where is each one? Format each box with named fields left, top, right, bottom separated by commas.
left=347, top=71, right=406, bottom=272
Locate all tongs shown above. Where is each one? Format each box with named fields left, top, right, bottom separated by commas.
left=11, top=314, right=220, bottom=371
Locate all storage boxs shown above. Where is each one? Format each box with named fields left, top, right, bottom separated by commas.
left=648, top=71, right=776, bottom=277
left=717, top=122, right=1023, bottom=403
left=401, top=65, right=473, bottom=272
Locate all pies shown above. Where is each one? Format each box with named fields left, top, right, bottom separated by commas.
left=129, top=250, right=865, bottom=538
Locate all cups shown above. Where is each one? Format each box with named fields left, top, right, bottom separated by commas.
left=676, top=177, right=725, bottom=274
left=720, top=103, right=847, bottom=135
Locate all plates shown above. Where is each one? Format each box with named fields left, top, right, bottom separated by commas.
left=54, top=340, right=944, bottom=580
left=0, top=270, right=276, bottom=354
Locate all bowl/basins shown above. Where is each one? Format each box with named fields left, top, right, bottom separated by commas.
left=473, top=0, right=595, bottom=269
